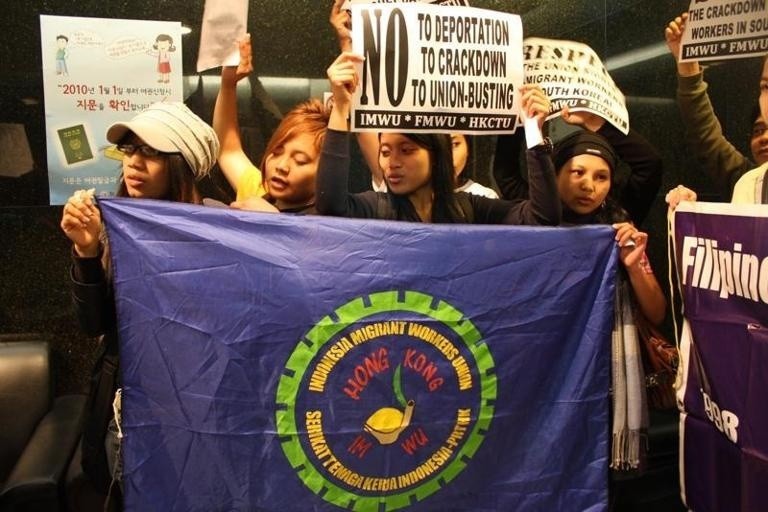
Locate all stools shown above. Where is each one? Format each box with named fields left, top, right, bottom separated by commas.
left=608, top=409, right=684, bottom=510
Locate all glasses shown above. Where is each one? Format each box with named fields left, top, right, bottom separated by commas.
left=116, top=143, right=162, bottom=157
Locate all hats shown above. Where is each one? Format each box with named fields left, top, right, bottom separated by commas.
left=105, top=100, right=221, bottom=182
left=552, top=130, right=618, bottom=173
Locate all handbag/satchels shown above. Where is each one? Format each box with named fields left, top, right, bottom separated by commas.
left=640, top=327, right=680, bottom=413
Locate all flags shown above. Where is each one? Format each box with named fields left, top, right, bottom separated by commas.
left=93, top=197, right=618, bottom=511
left=666, top=198, right=767, bottom=511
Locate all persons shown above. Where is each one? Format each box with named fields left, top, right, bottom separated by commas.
left=210, top=35, right=330, bottom=218
left=664, top=10, right=767, bottom=190
left=535, top=106, right=666, bottom=486
left=62, top=101, right=220, bottom=511
left=330, top=0, right=498, bottom=199
left=668, top=51, right=768, bottom=204
left=314, top=52, right=562, bottom=225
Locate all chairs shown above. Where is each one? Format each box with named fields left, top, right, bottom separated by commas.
left=0, top=338, right=97, bottom=510
